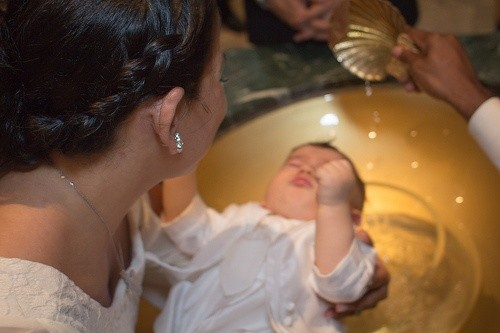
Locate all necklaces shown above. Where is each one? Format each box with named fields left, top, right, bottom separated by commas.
left=49, top=166, right=143, bottom=302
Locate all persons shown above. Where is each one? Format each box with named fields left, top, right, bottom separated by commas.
left=219, top=0, right=419, bottom=59
left=392, top=26, right=500, bottom=172
left=153, top=140, right=378, bottom=331
left=0, top=0, right=392, bottom=332
